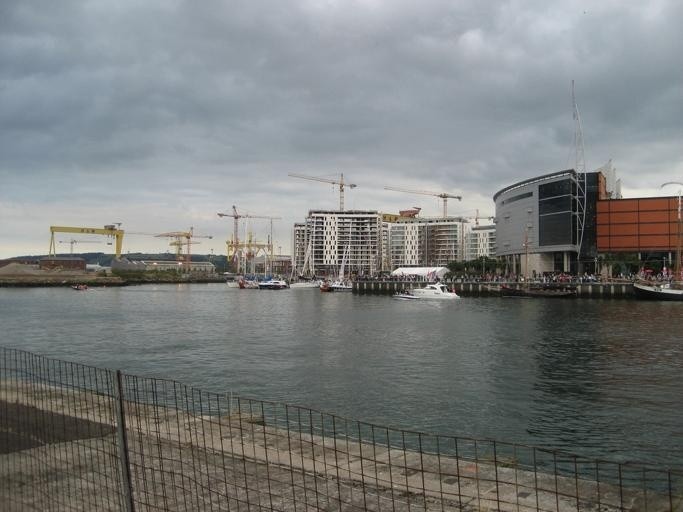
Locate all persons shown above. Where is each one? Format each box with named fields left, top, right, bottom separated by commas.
left=478, top=267, right=674, bottom=286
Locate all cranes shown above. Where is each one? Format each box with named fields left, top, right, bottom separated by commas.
left=217, top=204, right=281, bottom=276
left=48, top=222, right=124, bottom=258
left=468, top=209, right=493, bottom=224
left=154, top=226, right=213, bottom=264
left=288, top=171, right=357, bottom=211
left=385, top=184, right=463, bottom=218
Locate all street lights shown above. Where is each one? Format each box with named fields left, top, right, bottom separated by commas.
left=594, top=258, right=597, bottom=276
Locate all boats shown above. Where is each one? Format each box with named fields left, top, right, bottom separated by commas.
left=228, top=276, right=290, bottom=289
left=499, top=284, right=578, bottom=296
left=633, top=281, right=683, bottom=297
left=290, top=280, right=353, bottom=291
left=72, top=284, right=85, bottom=290
left=392, top=282, right=461, bottom=301
